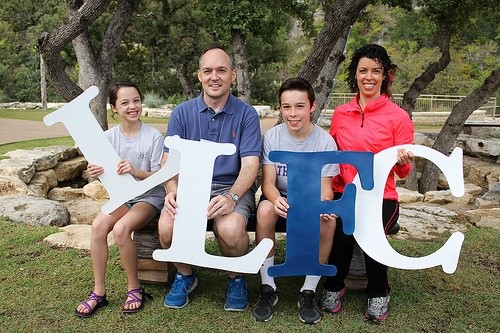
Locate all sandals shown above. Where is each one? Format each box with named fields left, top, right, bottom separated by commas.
left=75, top=290, right=108, bottom=317
left=122, top=287, right=144, bottom=313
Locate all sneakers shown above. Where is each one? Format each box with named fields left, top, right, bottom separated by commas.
left=164, top=271, right=198, bottom=308
left=224, top=276, right=249, bottom=311
left=251, top=284, right=278, bottom=322
left=365, top=296, right=391, bottom=320
left=320, top=287, right=345, bottom=312
left=297, top=290, right=321, bottom=323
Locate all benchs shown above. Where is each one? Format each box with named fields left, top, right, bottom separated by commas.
left=463, top=121, right=500, bottom=135
left=128, top=206, right=369, bottom=291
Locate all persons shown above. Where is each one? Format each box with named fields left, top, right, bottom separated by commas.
left=75, top=80, right=165, bottom=318
left=319, top=44, right=415, bottom=321
left=251, top=78, right=341, bottom=325
left=157, top=48, right=262, bottom=311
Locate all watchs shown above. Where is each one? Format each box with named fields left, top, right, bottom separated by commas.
left=226, top=191, right=240, bottom=205
